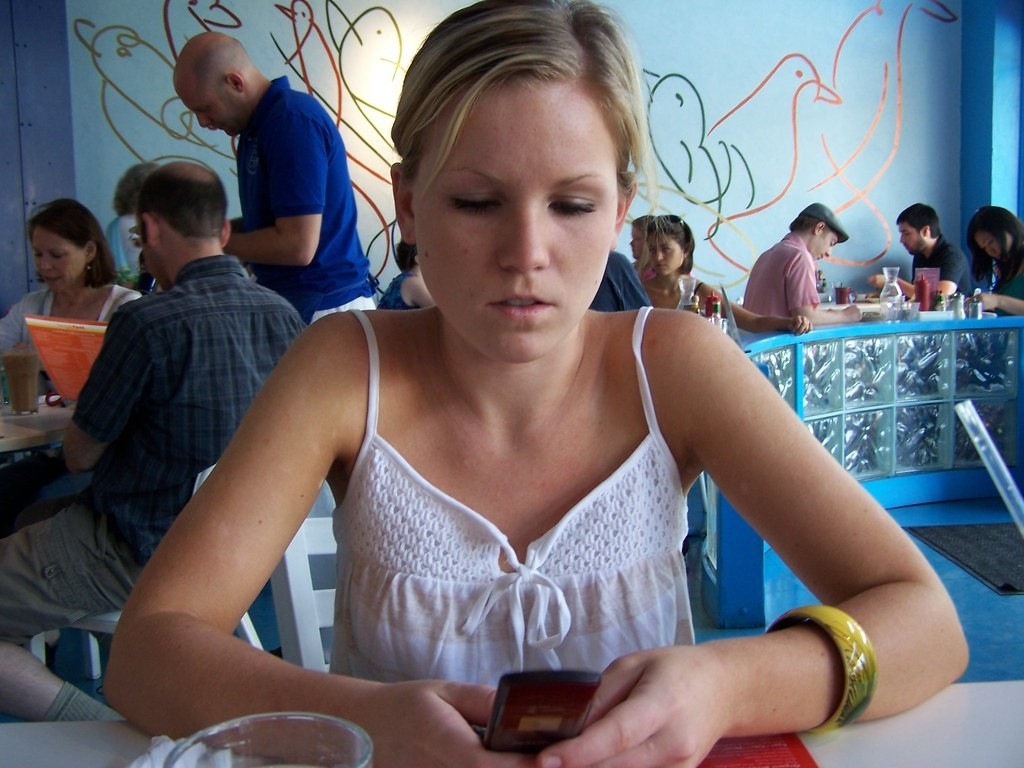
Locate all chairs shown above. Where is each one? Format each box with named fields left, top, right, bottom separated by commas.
left=272, top=506, right=346, bottom=671
left=14, top=448, right=271, bottom=682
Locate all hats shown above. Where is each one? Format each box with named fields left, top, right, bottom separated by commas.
left=798, top=203, right=849, bottom=243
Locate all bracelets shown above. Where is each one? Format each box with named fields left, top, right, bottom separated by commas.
left=766, top=605, right=880, bottom=734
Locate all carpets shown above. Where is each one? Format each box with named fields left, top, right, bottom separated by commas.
left=903, top=523, right=1024, bottom=595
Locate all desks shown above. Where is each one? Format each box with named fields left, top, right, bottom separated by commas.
left=823, top=293, right=997, bottom=321
left=0, top=400, right=81, bottom=459
left=3, top=678, right=1023, bottom=768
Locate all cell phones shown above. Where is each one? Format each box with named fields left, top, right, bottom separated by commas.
left=482, top=669, right=601, bottom=752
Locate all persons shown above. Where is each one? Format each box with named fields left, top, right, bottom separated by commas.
left=867, top=203, right=974, bottom=297
left=104, top=0, right=970, bottom=768
left=967, top=206, right=1024, bottom=315
left=590, top=215, right=816, bottom=335
left=0, top=32, right=438, bottom=722
left=742, top=203, right=862, bottom=325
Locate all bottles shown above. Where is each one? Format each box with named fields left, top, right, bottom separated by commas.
left=915, top=275, right=933, bottom=312
left=821, top=279, right=828, bottom=293
left=688, top=297, right=730, bottom=335
left=816, top=270, right=823, bottom=293
left=880, top=268, right=901, bottom=297
left=934, top=296, right=947, bottom=312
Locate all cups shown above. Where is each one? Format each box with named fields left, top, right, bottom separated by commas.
left=836, top=287, right=856, bottom=305
left=904, top=302, right=920, bottom=321
left=2, top=348, right=40, bottom=414
left=881, top=295, right=905, bottom=321
left=163, top=711, right=373, bottom=767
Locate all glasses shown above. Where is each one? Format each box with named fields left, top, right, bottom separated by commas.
left=128, top=227, right=146, bottom=246
left=652, top=215, right=685, bottom=229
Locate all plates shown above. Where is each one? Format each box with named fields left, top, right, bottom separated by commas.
left=865, top=298, right=880, bottom=302
